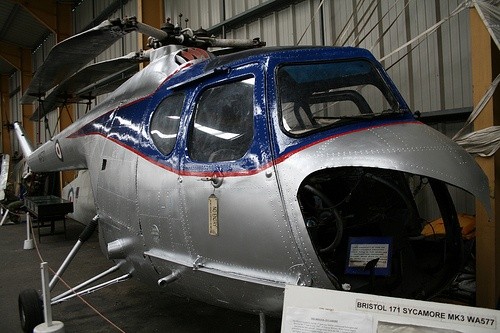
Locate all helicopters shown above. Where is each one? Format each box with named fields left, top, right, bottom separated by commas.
left=0, top=0, right=499, bottom=333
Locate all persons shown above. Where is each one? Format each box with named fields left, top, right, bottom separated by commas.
left=220, top=96, right=258, bottom=160
